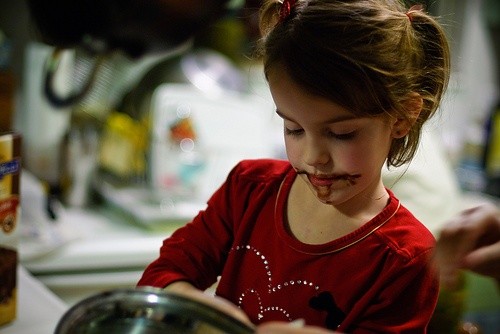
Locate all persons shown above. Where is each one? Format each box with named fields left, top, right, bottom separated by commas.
left=439, top=201, right=499, bottom=286
left=136, top=1, right=442, bottom=334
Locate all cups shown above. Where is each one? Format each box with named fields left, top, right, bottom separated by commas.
left=0, top=247, right=18, bottom=327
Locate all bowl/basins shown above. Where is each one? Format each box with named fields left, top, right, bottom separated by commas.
left=52, top=290, right=262, bottom=334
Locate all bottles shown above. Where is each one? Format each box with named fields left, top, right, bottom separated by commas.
left=0, top=129, right=23, bottom=240
